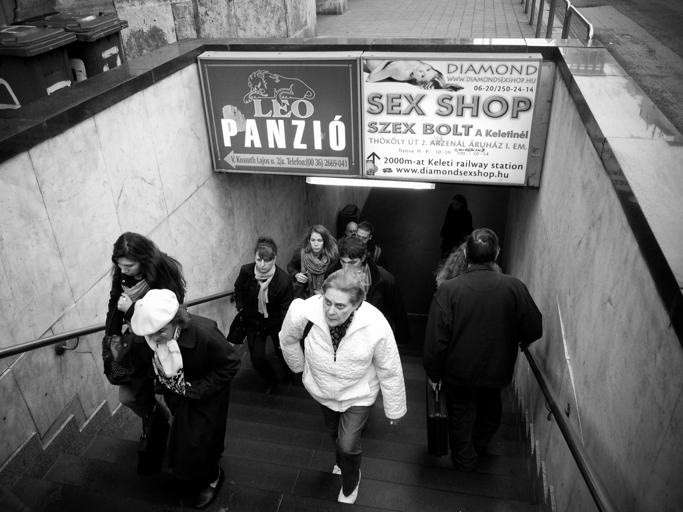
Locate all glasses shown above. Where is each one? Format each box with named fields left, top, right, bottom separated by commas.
left=145, top=332, right=169, bottom=342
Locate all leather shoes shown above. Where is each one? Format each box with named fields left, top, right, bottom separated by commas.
left=191, top=464, right=225, bottom=510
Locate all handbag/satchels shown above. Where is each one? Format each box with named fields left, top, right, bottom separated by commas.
left=225, top=308, right=249, bottom=346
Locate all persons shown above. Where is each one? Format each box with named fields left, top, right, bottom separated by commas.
left=324, top=237, right=398, bottom=322
left=364, top=58, right=464, bottom=92
left=287, top=224, right=340, bottom=301
left=235, top=237, right=296, bottom=396
left=278, top=264, right=409, bottom=504
left=421, top=227, right=544, bottom=482
left=115, top=287, right=242, bottom=512
left=100, top=231, right=186, bottom=440
left=337, top=191, right=504, bottom=287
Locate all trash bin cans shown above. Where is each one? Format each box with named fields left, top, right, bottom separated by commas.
left=0, top=11, right=128, bottom=104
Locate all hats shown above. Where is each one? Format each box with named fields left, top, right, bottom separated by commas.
left=130, top=288, right=180, bottom=338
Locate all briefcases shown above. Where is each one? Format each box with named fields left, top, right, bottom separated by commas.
left=424, top=373, right=452, bottom=459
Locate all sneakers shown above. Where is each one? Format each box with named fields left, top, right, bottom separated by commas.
left=333, top=462, right=363, bottom=505
left=137, top=415, right=169, bottom=480
